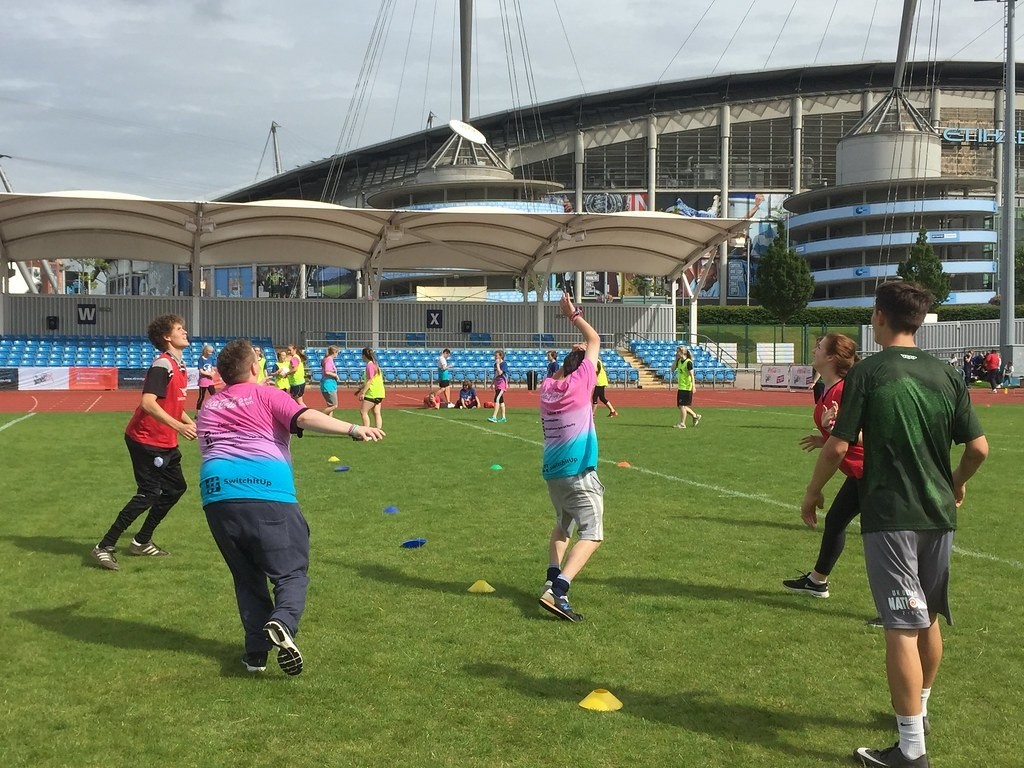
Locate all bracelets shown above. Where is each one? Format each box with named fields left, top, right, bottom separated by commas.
left=570, top=307, right=582, bottom=324
left=824, top=420, right=835, bottom=429
left=349, top=424, right=359, bottom=437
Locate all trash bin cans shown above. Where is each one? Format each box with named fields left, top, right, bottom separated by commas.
left=527, top=371, right=538, bottom=390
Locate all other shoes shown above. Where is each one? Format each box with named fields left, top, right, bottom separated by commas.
left=487, top=416, right=497, bottom=423
left=607, top=410, right=618, bottom=417
left=497, top=417, right=507, bottom=422
left=447, top=402, right=456, bottom=408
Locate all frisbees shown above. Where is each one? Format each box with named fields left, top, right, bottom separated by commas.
left=448, top=120, right=487, bottom=144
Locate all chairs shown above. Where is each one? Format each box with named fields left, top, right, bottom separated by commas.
left=304, top=333, right=735, bottom=381
left=0, top=334, right=282, bottom=380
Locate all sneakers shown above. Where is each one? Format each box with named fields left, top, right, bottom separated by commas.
left=672, top=423, right=686, bottom=428
left=541, top=579, right=583, bottom=623
left=129, top=537, right=171, bottom=556
left=867, top=616, right=885, bottom=628
left=243, top=653, right=266, bottom=672
left=853, top=739, right=928, bottom=768
left=693, top=414, right=701, bottom=426
left=263, top=619, right=303, bottom=676
left=782, top=569, right=829, bottom=598
left=892, top=699, right=931, bottom=735
left=90, top=542, right=119, bottom=571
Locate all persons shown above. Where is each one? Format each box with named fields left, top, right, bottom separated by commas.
left=459, top=380, right=481, bottom=409
left=253, top=343, right=306, bottom=406
left=782, top=334, right=884, bottom=628
left=592, top=359, right=619, bottom=418
left=423, top=392, right=440, bottom=409
left=487, top=350, right=509, bottom=423
left=353, top=347, right=385, bottom=429
left=539, top=293, right=605, bottom=622
left=320, top=344, right=340, bottom=416
left=197, top=338, right=386, bottom=676
left=801, top=282, right=989, bottom=768
left=597, top=291, right=613, bottom=303
left=809, top=338, right=825, bottom=404
left=91, top=314, right=197, bottom=571
left=671, top=346, right=701, bottom=428
left=948, top=349, right=1014, bottom=394
left=193, top=345, right=217, bottom=421
left=547, top=351, right=559, bottom=378
left=437, top=349, right=455, bottom=408
